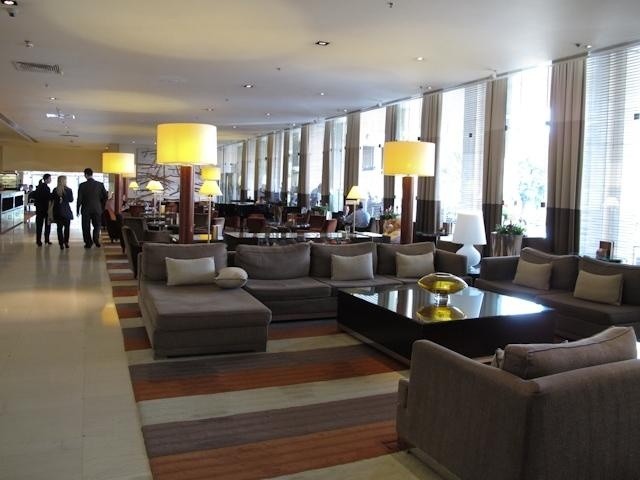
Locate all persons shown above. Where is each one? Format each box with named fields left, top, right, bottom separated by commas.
left=105, top=192, right=115, bottom=211
left=344, top=202, right=370, bottom=231
left=338, top=205, right=352, bottom=218
left=19, top=184, right=33, bottom=203
left=77, top=167, right=108, bottom=248
left=247, top=196, right=252, bottom=202
left=32, top=174, right=52, bottom=246
left=52, top=175, right=74, bottom=249
left=256, top=196, right=267, bottom=205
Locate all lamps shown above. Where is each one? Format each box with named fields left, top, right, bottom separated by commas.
left=128, top=181, right=140, bottom=197
left=453, top=213, right=487, bottom=273
left=347, top=186, right=370, bottom=232
left=156, top=121, right=217, bottom=243
left=201, top=164, right=222, bottom=242
left=99, top=152, right=141, bottom=214
left=384, top=140, right=436, bottom=243
left=145, top=181, right=166, bottom=209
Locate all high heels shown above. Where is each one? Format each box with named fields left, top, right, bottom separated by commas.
left=37, top=240, right=42, bottom=248
left=45, top=241, right=52, bottom=244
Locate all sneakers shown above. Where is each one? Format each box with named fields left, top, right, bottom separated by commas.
left=59, top=240, right=64, bottom=249
left=63, top=239, right=69, bottom=248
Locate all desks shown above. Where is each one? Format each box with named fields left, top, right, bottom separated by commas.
left=227, top=229, right=392, bottom=248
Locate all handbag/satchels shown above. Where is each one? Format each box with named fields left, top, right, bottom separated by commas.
left=48, top=199, right=54, bottom=226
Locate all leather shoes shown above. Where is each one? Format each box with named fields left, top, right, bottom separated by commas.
left=85, top=240, right=93, bottom=248
left=94, top=239, right=101, bottom=247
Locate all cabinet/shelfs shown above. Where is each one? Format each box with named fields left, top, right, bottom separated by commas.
left=0, top=190, right=25, bottom=235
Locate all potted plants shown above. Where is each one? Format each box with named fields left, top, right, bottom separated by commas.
left=490, top=221, right=524, bottom=256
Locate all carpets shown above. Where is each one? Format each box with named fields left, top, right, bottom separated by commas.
left=102, top=225, right=504, bottom=480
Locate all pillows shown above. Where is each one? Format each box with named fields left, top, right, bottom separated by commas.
left=515, top=245, right=640, bottom=312
left=491, top=340, right=568, bottom=368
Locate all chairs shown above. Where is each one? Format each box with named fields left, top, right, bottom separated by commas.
left=25, top=194, right=36, bottom=211
left=102, top=195, right=375, bottom=280
left=396, top=325, right=640, bottom=480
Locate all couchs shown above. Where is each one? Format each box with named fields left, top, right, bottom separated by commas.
left=474, top=247, right=640, bottom=342
left=137, top=240, right=472, bottom=360
